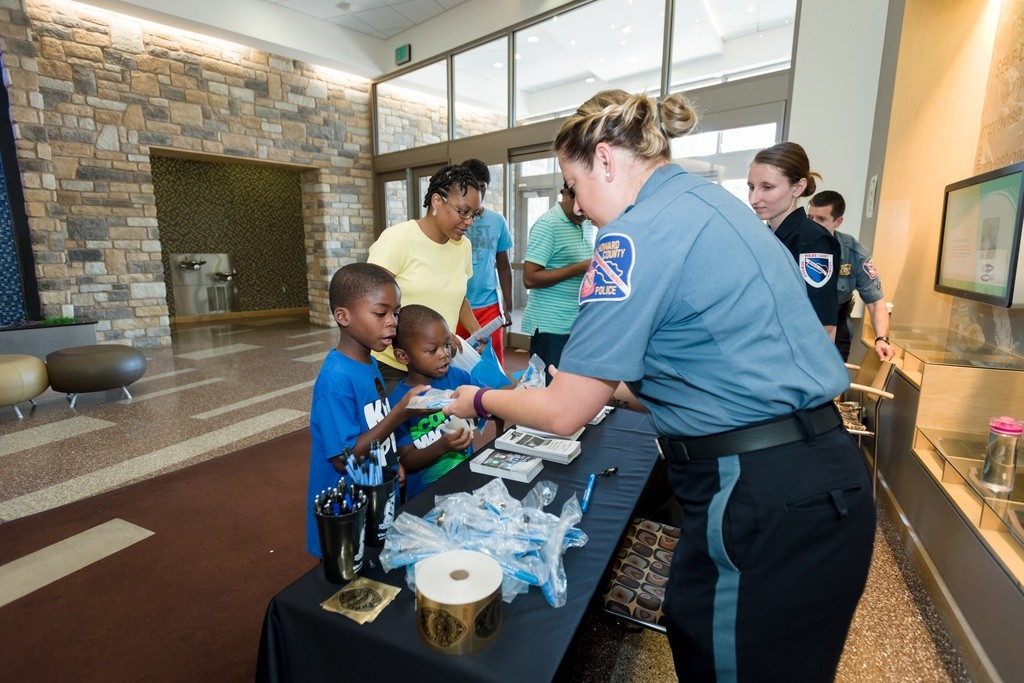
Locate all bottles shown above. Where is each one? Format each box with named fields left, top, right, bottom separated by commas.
left=980, top=415, right=1024, bottom=492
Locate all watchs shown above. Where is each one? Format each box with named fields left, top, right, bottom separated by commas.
left=875, top=337, right=891, bottom=345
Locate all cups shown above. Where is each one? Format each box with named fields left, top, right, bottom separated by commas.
left=349, top=466, right=400, bottom=547
left=313, top=491, right=369, bottom=584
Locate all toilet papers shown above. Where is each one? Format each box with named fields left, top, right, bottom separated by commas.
left=414, top=548, right=505, bottom=656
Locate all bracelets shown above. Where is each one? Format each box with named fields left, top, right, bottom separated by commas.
left=473, top=388, right=495, bottom=420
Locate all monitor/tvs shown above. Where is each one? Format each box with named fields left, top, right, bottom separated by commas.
left=934, top=160, right=1024, bottom=308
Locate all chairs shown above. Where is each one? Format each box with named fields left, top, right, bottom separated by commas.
left=834, top=347, right=898, bottom=504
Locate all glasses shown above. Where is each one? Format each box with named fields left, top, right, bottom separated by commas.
left=441, top=196, right=482, bottom=222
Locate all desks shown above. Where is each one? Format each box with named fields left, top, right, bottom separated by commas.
left=254, top=405, right=659, bottom=683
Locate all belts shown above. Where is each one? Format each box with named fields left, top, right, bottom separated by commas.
left=654, top=405, right=843, bottom=461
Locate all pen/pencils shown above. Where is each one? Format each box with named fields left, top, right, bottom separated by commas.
left=581, top=473, right=598, bottom=513
left=314, top=441, right=383, bottom=516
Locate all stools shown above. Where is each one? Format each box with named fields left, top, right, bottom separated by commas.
left=44, top=343, right=149, bottom=409
left=0, top=353, right=49, bottom=420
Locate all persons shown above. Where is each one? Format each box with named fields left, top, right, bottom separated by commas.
left=388, top=304, right=516, bottom=508
left=520, top=182, right=593, bottom=388
left=807, top=190, right=896, bottom=366
left=746, top=142, right=842, bottom=350
left=367, top=165, right=481, bottom=399
left=442, top=90, right=877, bottom=683
left=455, top=158, right=512, bottom=437
left=306, top=263, right=438, bottom=562
